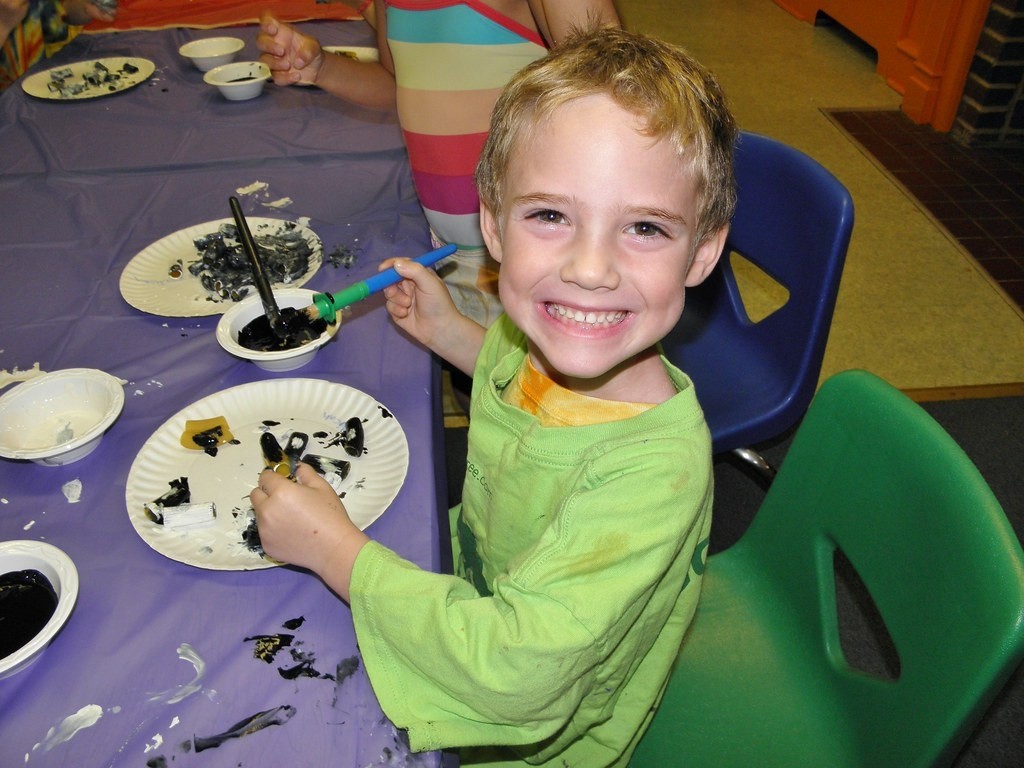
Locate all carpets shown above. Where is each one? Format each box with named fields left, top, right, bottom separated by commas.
left=447, top=381, right=1024, bottom=768
left=820, top=105, right=1024, bottom=318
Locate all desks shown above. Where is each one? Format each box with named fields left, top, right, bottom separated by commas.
left=0, top=0, right=442, bottom=768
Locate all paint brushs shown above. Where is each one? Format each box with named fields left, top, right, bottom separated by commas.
left=225, top=194, right=293, bottom=348
left=282, top=239, right=462, bottom=341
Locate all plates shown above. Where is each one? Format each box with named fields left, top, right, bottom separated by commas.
left=22, top=58, right=155, bottom=99
left=125, top=378, right=408, bottom=570
left=322, top=46, right=379, bottom=62
left=119, top=217, right=323, bottom=317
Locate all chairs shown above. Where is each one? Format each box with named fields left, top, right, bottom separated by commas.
left=629, top=367, right=1024, bottom=768
left=659, top=131, right=854, bottom=490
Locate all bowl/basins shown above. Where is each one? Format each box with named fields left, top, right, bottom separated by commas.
left=204, top=62, right=270, bottom=101
left=179, top=37, right=245, bottom=72
left=216, top=289, right=342, bottom=372
left=0, top=540, right=78, bottom=673
left=0, top=368, right=125, bottom=466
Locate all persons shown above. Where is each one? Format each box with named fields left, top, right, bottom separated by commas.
left=249, top=11, right=740, bottom=768
left=0, top=0, right=117, bottom=95
left=259, top=1, right=626, bottom=419
